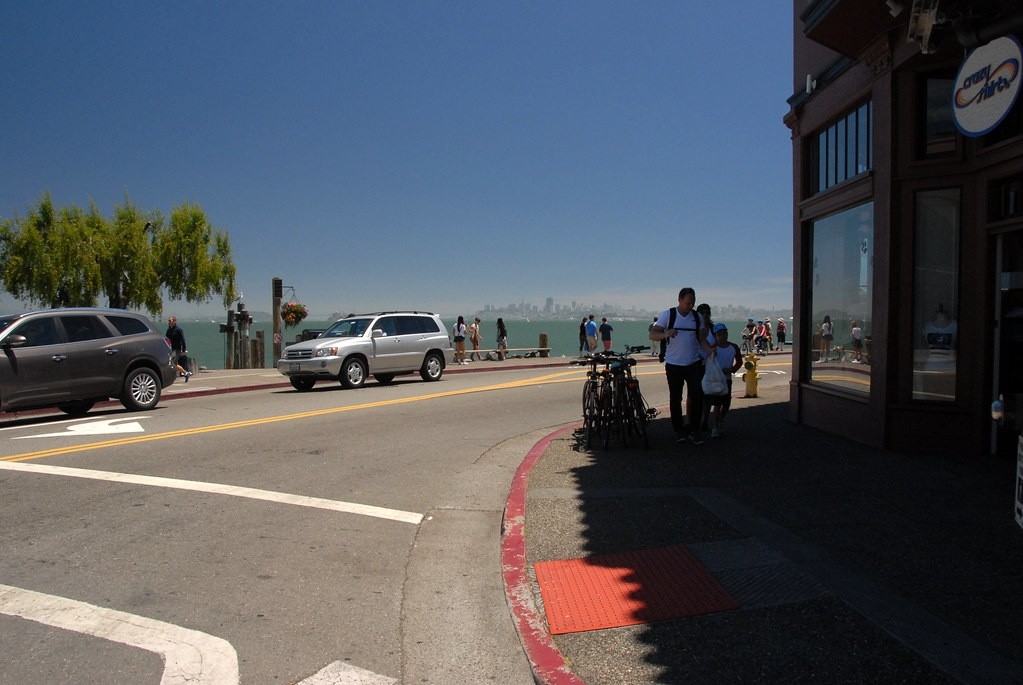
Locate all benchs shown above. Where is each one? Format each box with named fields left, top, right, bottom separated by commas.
left=454, top=347, right=552, bottom=363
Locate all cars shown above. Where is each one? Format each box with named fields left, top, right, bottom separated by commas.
left=0, top=308, right=177, bottom=418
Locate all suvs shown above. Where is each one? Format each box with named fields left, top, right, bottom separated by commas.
left=277, top=310, right=455, bottom=390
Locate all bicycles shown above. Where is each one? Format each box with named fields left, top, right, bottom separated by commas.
left=573, top=346, right=659, bottom=450
left=739, top=332, right=769, bottom=357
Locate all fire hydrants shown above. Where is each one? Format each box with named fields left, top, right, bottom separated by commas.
left=742, top=354, right=762, bottom=399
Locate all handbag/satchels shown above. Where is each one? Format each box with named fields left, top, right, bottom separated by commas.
left=701, top=353, right=729, bottom=396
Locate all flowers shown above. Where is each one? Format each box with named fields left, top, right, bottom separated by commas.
left=281, top=303, right=308, bottom=330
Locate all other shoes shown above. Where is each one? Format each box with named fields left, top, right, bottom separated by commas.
left=470, top=356, right=476, bottom=362
left=458, top=359, right=466, bottom=365
left=718, top=423, right=725, bottom=434
left=688, top=430, right=704, bottom=445
left=671, top=431, right=686, bottom=443
left=710, top=427, right=720, bottom=439
left=184, top=372, right=190, bottom=383
left=815, top=358, right=829, bottom=364
left=852, top=359, right=863, bottom=364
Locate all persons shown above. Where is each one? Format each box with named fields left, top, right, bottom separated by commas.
left=599, top=318, right=613, bottom=358
left=469, top=317, right=482, bottom=361
left=920, top=311, right=957, bottom=400
left=453, top=316, right=469, bottom=365
left=585, top=315, right=599, bottom=356
left=741, top=317, right=774, bottom=353
left=776, top=318, right=787, bottom=352
left=649, top=287, right=717, bottom=445
left=650, top=317, right=659, bottom=356
left=166, top=315, right=190, bottom=383
left=815, top=315, right=834, bottom=363
left=850, top=321, right=864, bottom=363
left=702, top=323, right=743, bottom=437
left=496, top=318, right=507, bottom=360
left=579, top=317, right=589, bottom=357
left=684, top=304, right=717, bottom=434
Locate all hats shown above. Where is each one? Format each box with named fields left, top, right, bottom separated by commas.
left=713, top=323, right=728, bottom=336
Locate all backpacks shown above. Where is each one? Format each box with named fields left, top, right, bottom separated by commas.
left=658, top=308, right=701, bottom=362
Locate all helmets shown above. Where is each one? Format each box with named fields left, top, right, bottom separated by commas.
left=757, top=319, right=764, bottom=323
left=747, top=318, right=753, bottom=321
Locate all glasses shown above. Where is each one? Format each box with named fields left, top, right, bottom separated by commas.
left=698, top=310, right=711, bottom=315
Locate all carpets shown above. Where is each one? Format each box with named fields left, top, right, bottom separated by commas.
left=534, top=546, right=742, bottom=635
